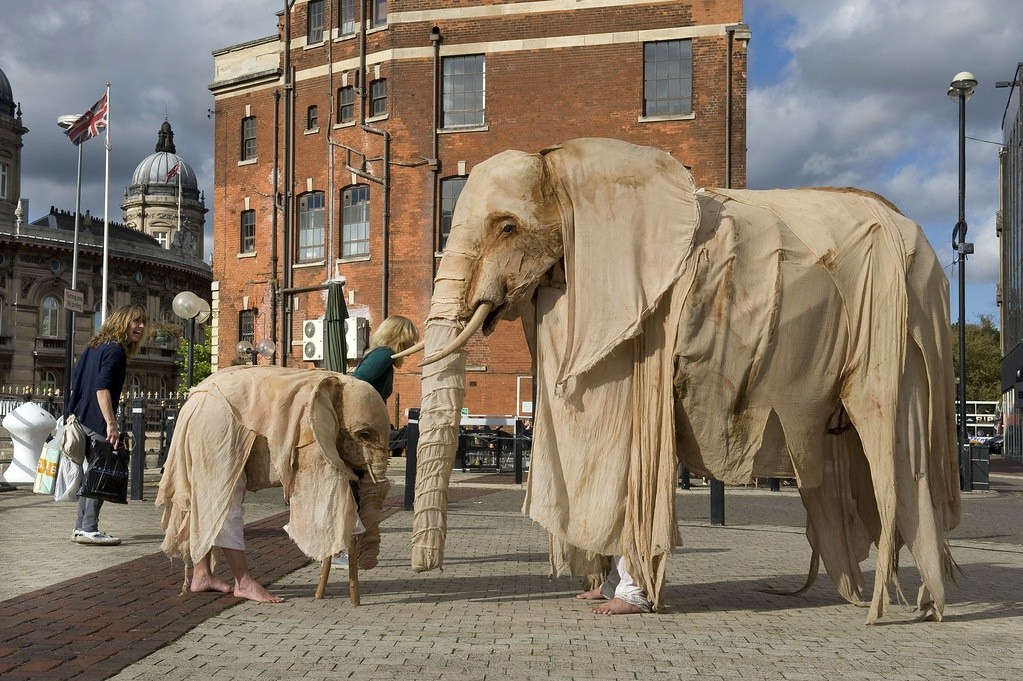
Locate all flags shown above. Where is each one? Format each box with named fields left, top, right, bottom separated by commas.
left=165, top=163, right=179, bottom=183
left=62, top=93, right=107, bottom=146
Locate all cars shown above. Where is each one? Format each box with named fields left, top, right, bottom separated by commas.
left=985, top=434, right=1004, bottom=453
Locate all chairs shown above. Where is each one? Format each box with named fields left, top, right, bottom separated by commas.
left=455, top=428, right=533, bottom=473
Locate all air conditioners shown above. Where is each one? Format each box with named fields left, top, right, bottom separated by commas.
left=302, top=317, right=367, bottom=361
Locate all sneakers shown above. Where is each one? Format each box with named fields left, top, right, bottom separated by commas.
left=69, top=529, right=121, bottom=546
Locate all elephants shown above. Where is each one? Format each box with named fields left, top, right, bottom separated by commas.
left=411, top=136, right=964, bottom=633
left=153, top=364, right=391, bottom=607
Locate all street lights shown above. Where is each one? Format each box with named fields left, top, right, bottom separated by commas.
left=944, top=71, right=981, bottom=495
left=56, top=112, right=107, bottom=427
left=169, top=289, right=213, bottom=392
left=233, top=338, right=276, bottom=366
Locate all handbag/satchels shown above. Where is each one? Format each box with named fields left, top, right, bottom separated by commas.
left=32, top=424, right=87, bottom=501
left=75, top=431, right=137, bottom=505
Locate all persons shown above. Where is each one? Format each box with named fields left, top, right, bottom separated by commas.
left=576, top=552, right=653, bottom=614
left=319, top=314, right=419, bottom=568
left=191, top=404, right=285, bottom=604
left=63, top=304, right=148, bottom=546
